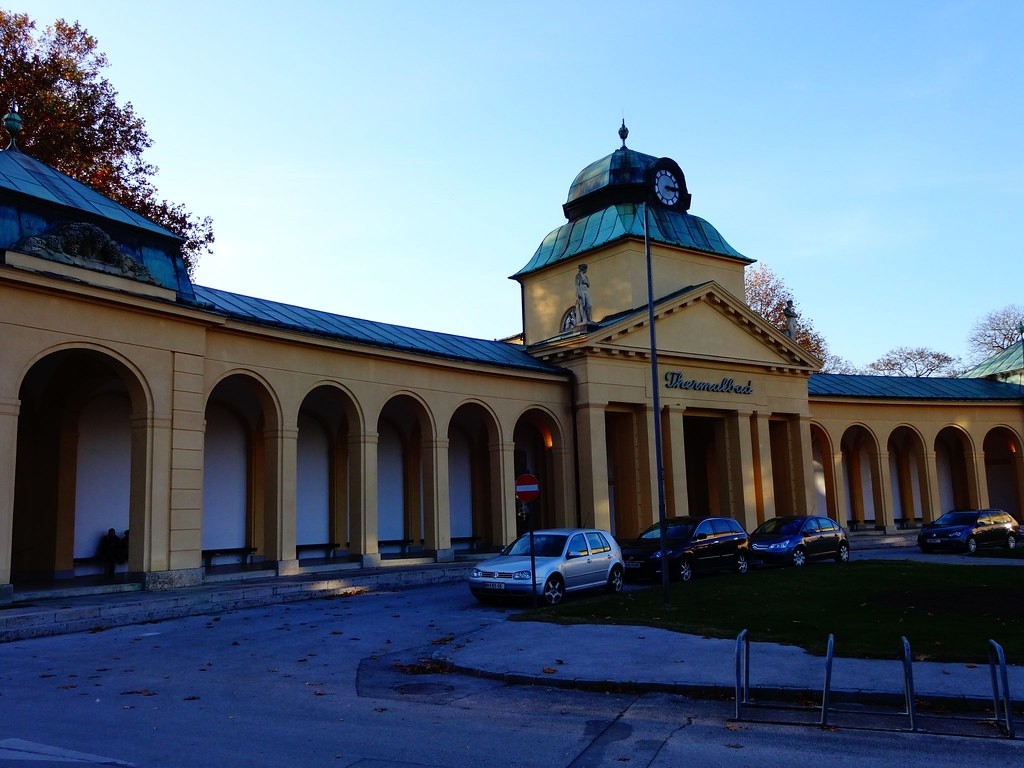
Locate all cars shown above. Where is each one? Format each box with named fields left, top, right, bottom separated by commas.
left=469, top=527, right=626, bottom=607
left=748, top=514, right=851, bottom=568
left=916, top=507, right=1022, bottom=557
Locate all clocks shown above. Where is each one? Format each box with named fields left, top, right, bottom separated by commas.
left=655, top=170, right=680, bottom=206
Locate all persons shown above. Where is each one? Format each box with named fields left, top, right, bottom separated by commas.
left=575, top=263, right=592, bottom=324
left=95, top=528, right=130, bottom=577
left=785, top=300, right=798, bottom=341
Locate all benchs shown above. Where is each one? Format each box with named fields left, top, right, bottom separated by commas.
left=74, top=557, right=128, bottom=575
left=847, top=520, right=860, bottom=531
left=864, top=519, right=910, bottom=528
left=914, top=518, right=923, bottom=522
left=296, top=543, right=341, bottom=560
left=202, top=548, right=259, bottom=571
left=421, top=536, right=484, bottom=550
left=347, top=540, right=414, bottom=555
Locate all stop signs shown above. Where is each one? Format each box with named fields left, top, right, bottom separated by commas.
left=515, top=473, right=540, bottom=502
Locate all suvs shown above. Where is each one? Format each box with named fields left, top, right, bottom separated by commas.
left=621, top=515, right=752, bottom=586
